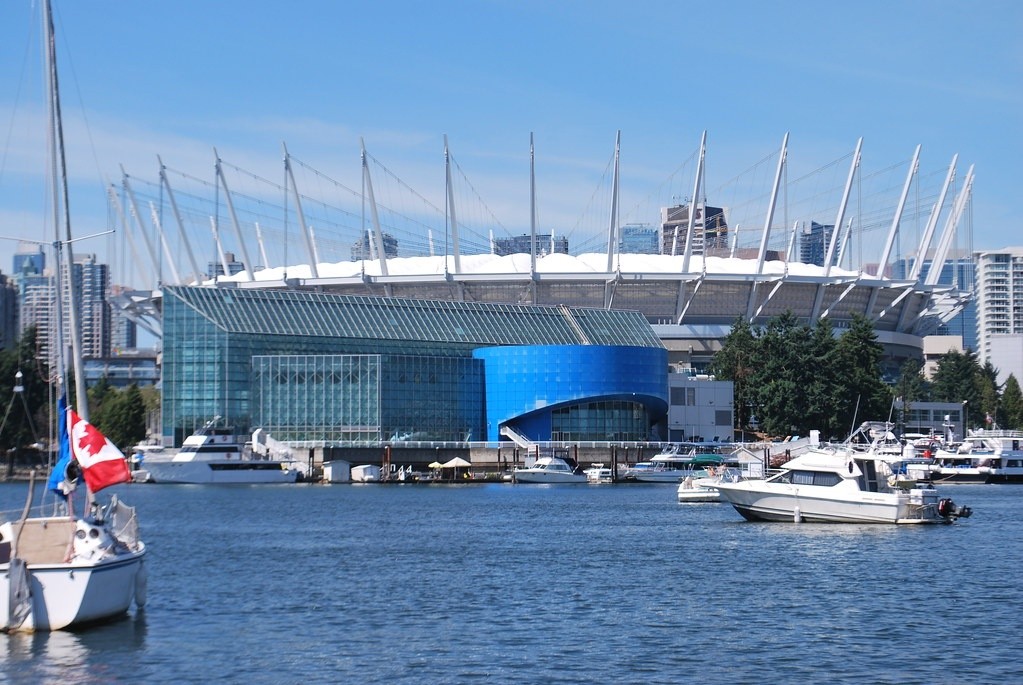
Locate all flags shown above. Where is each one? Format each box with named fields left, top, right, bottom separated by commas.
left=50, top=395, right=130, bottom=499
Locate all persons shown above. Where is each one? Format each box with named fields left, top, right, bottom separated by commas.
left=135, top=450, right=144, bottom=467
left=707, top=464, right=715, bottom=477
left=717, top=464, right=726, bottom=480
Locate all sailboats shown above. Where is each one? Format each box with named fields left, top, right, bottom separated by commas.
left=0, top=0, right=152, bottom=640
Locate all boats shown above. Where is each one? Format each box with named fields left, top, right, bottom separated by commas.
left=583, top=463, right=611, bottom=482
left=905, top=428, right=1023, bottom=485
left=514, top=456, right=588, bottom=483
left=651, top=439, right=730, bottom=471
left=132, top=416, right=300, bottom=484
left=842, top=393, right=904, bottom=459
left=626, top=444, right=720, bottom=483
left=700, top=448, right=975, bottom=527
left=676, top=465, right=738, bottom=504
